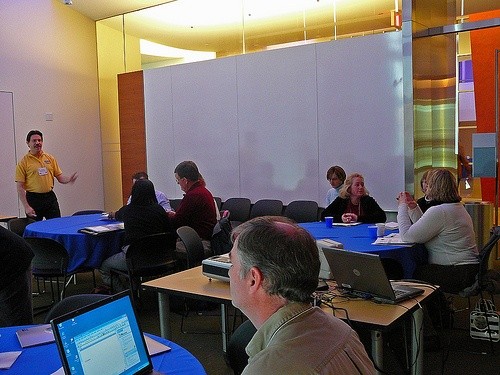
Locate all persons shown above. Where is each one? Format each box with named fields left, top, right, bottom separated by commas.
left=416, top=171, right=444, bottom=214
left=228, top=216, right=376, bottom=375
left=127, top=172, right=171, bottom=213
left=325, top=166, right=346, bottom=209
left=99, top=179, right=178, bottom=293
left=169, top=161, right=217, bottom=259
left=14, top=129, right=80, bottom=222
left=394, top=167, right=479, bottom=351
left=320, top=172, right=387, bottom=224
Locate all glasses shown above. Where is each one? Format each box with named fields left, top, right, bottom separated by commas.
left=175, top=176, right=184, bottom=184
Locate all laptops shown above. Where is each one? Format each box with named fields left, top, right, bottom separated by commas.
left=321, top=247, right=425, bottom=304
left=50, top=288, right=162, bottom=375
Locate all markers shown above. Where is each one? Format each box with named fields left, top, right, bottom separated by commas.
left=32, top=214, right=37, bottom=217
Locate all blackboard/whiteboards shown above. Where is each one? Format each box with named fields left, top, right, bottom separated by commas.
left=0, top=91, right=20, bottom=220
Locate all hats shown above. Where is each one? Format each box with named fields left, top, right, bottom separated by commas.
left=174, top=161, right=202, bottom=181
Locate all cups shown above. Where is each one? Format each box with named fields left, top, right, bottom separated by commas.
left=325, top=217, right=333, bottom=228
left=375, top=223, right=385, bottom=236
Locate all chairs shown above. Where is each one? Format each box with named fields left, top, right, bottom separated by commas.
left=7, top=219, right=69, bottom=303
left=284, top=200, right=319, bottom=223
left=443, top=226, right=500, bottom=345
left=250, top=200, right=283, bottom=221
left=109, top=231, right=177, bottom=322
left=221, top=198, right=251, bottom=228
left=176, top=225, right=238, bottom=335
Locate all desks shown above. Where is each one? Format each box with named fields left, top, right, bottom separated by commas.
left=23, top=213, right=125, bottom=288
left=298, top=223, right=422, bottom=278
left=140, top=266, right=440, bottom=375
left=0, top=325, right=206, bottom=375
